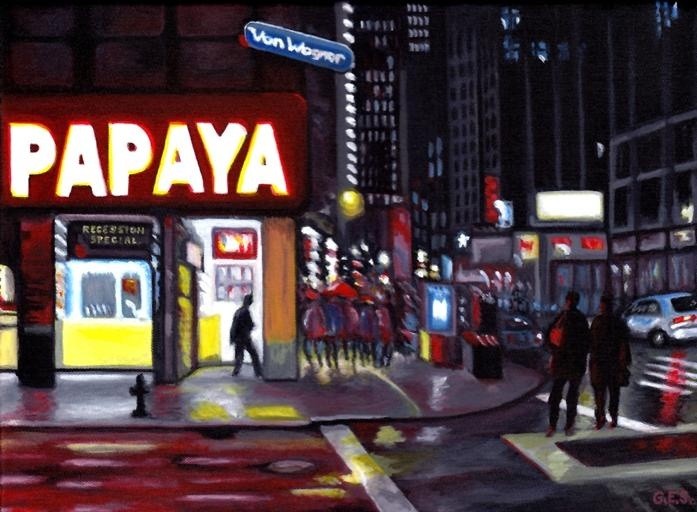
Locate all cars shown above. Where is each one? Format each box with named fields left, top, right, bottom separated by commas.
left=621, top=292, right=697, bottom=348
left=499, top=312, right=544, bottom=354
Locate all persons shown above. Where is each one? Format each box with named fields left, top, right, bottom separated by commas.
left=303, top=290, right=398, bottom=366
left=230, top=295, right=263, bottom=376
left=589, top=297, right=633, bottom=431
left=543, top=291, right=590, bottom=437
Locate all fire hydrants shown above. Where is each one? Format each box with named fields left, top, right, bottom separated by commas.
left=128, top=375, right=152, bottom=416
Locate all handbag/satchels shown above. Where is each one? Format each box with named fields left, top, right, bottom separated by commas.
left=549, top=314, right=568, bottom=348
